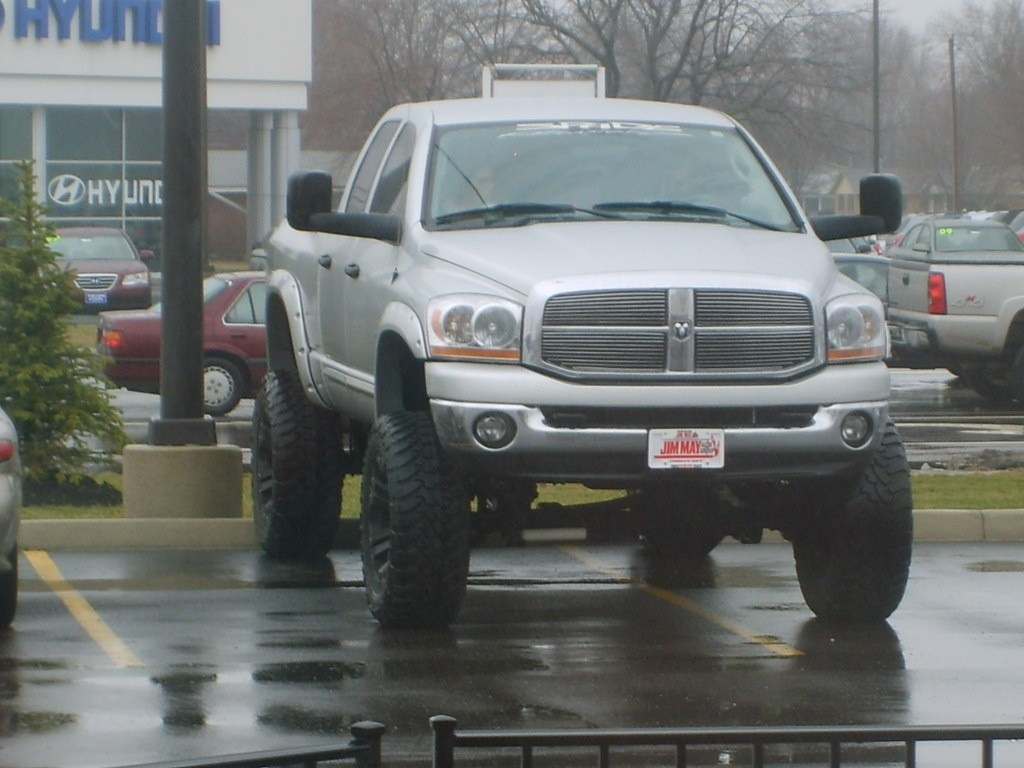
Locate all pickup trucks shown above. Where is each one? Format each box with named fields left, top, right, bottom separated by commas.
left=885, top=251, right=1024, bottom=408
left=248, top=59, right=913, bottom=626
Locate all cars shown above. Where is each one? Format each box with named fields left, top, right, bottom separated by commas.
left=825, top=208, right=1024, bottom=248
left=41, top=226, right=156, bottom=312
left=93, top=270, right=270, bottom=415
left=881, top=217, right=1024, bottom=263
